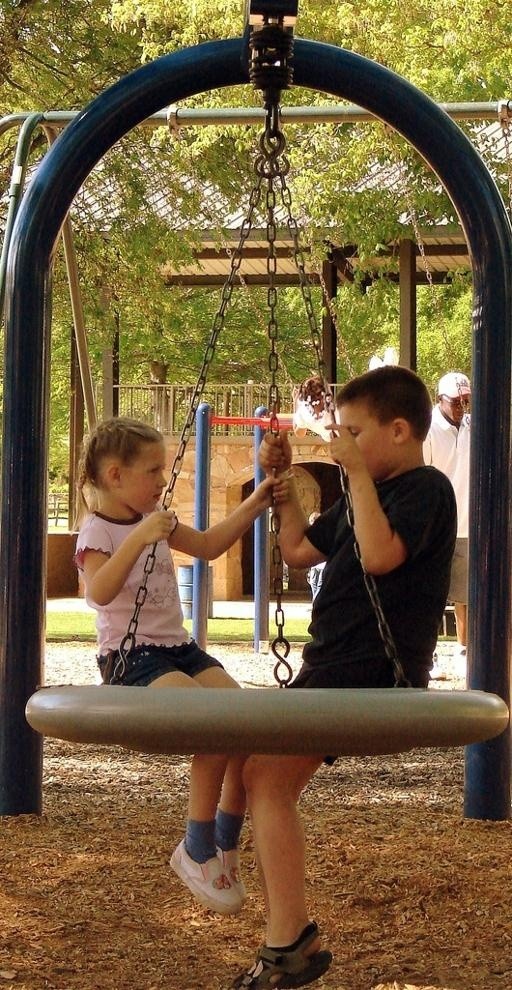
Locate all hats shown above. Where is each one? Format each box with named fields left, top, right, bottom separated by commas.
left=437, top=372, right=472, bottom=399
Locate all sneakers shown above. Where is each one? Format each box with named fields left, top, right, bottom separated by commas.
left=168, top=835, right=242, bottom=915
left=427, top=651, right=446, bottom=680
left=215, top=842, right=247, bottom=904
left=452, top=641, right=468, bottom=680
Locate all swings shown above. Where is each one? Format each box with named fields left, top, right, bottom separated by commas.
left=166, top=108, right=359, bottom=442
left=382, top=120, right=511, bottom=428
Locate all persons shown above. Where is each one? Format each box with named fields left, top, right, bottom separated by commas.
left=225, top=364, right=460, bottom=989
left=419, top=371, right=470, bottom=681
left=72, top=417, right=294, bottom=918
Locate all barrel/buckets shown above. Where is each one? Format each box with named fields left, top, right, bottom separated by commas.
left=178, top=565, right=213, bottom=620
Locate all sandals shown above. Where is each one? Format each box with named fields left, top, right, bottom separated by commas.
left=225, top=922, right=332, bottom=990
